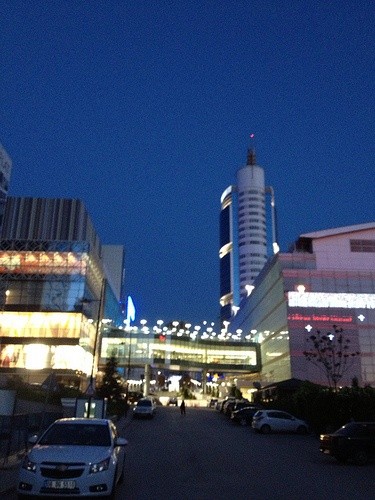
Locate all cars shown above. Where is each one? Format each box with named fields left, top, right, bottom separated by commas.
left=320, top=421, right=375, bottom=466
left=135, top=397, right=156, bottom=416
left=208, top=399, right=264, bottom=426
left=14, top=417, right=124, bottom=500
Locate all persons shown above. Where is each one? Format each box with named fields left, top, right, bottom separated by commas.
left=114, top=395, right=129, bottom=421
left=179, top=400, right=185, bottom=414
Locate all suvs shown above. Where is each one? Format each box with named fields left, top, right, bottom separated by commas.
left=252, top=410, right=309, bottom=435
